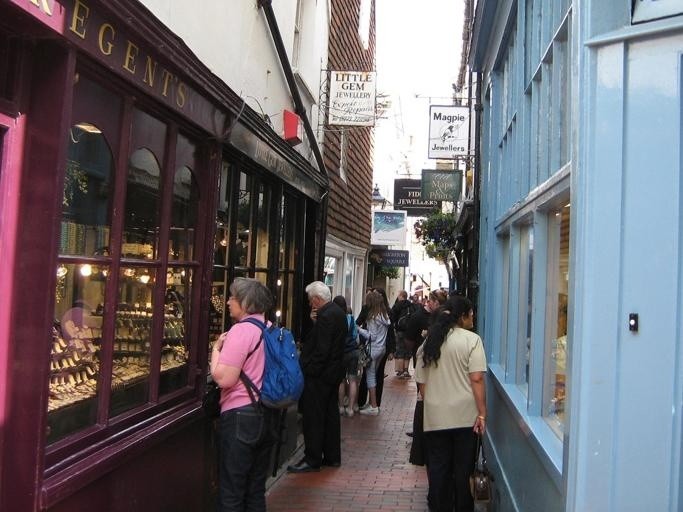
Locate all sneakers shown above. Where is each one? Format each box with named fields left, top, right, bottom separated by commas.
left=339, top=395, right=380, bottom=419
left=404, top=372, right=411, bottom=377
left=396, top=372, right=406, bottom=380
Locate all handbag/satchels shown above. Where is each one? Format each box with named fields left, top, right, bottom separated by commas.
left=469, top=425, right=493, bottom=504
left=358, top=335, right=373, bottom=369
left=202, top=380, right=222, bottom=419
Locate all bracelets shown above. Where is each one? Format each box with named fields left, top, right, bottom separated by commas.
left=211, top=348, right=219, bottom=352
left=477, top=414, right=485, bottom=420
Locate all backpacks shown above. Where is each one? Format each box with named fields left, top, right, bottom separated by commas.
left=238, top=318, right=306, bottom=411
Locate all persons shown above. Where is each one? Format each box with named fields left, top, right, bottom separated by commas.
left=333, top=286, right=449, bottom=416
left=61, top=289, right=96, bottom=369
left=412, top=294, right=489, bottom=512
left=209, top=276, right=274, bottom=512
left=285, top=279, right=348, bottom=474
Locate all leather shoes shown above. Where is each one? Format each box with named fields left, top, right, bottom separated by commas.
left=287, top=459, right=321, bottom=474
left=322, top=458, right=342, bottom=468
